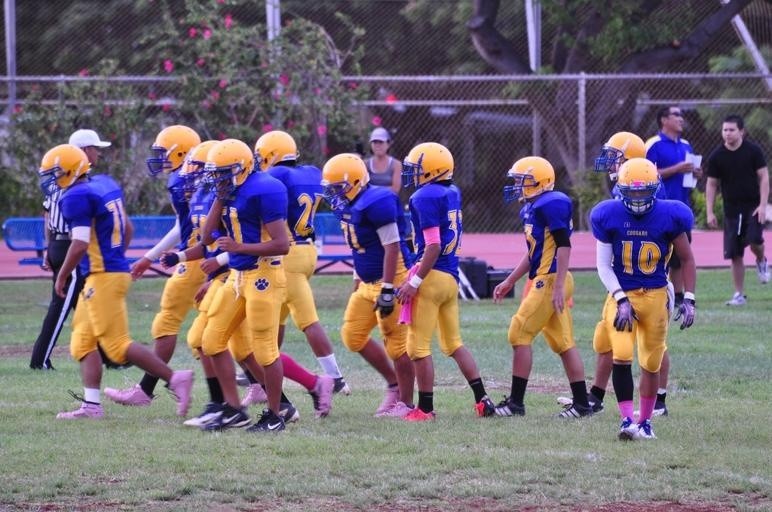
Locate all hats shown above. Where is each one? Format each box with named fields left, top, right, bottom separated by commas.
left=68, top=129, right=111, bottom=149
left=368, top=127, right=391, bottom=144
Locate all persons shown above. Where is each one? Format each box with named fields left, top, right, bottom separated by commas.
left=705, top=115, right=770, bottom=305
left=647, top=107, right=703, bottom=308
left=557, top=131, right=696, bottom=441
left=320, top=128, right=496, bottom=420
left=31, top=129, right=134, bottom=370
left=492, top=155, right=593, bottom=417
left=105, top=125, right=351, bottom=434
left=38, top=144, right=196, bottom=419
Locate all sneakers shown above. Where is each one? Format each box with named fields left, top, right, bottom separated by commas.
left=724, top=291, right=746, bottom=306
left=102, top=383, right=152, bottom=407
left=182, top=382, right=299, bottom=432
left=308, top=374, right=350, bottom=419
left=548, top=391, right=604, bottom=419
left=493, top=398, right=524, bottom=417
left=474, top=393, right=494, bottom=416
left=164, top=369, right=193, bottom=416
left=755, top=257, right=770, bottom=284
left=617, top=400, right=667, bottom=441
left=55, top=399, right=103, bottom=421
left=373, top=384, right=435, bottom=422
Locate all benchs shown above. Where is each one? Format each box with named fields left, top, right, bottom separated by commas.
left=3, top=213, right=415, bottom=276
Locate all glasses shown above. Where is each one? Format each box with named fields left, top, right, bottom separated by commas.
left=668, top=112, right=684, bottom=116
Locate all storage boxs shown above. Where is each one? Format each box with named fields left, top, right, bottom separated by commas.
left=457, top=252, right=489, bottom=299
left=486, top=268, right=516, bottom=299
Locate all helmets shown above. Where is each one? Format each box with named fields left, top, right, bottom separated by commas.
left=503, top=155, right=555, bottom=205
left=179, top=139, right=255, bottom=201
left=400, top=141, right=455, bottom=195
left=615, top=157, right=660, bottom=217
left=319, top=152, right=369, bottom=215
left=37, top=143, right=92, bottom=202
left=253, top=129, right=299, bottom=175
left=144, top=124, right=202, bottom=178
left=594, top=132, right=646, bottom=199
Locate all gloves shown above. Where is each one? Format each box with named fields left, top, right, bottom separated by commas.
left=612, top=288, right=640, bottom=334
left=372, top=282, right=394, bottom=318
left=162, top=250, right=187, bottom=268
left=673, top=291, right=696, bottom=330
